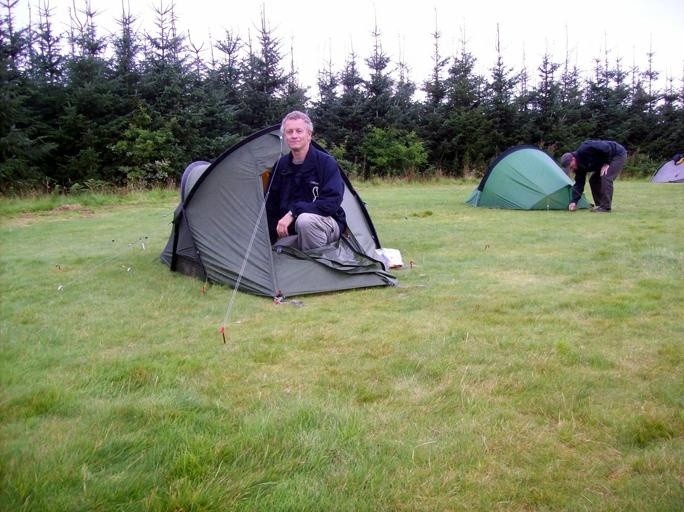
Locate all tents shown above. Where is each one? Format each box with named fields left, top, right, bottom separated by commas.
left=158, top=120, right=399, bottom=305
left=463, top=143, right=594, bottom=212
left=646, top=152, right=684, bottom=184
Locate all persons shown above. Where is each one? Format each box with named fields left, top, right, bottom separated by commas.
left=558, top=139, right=629, bottom=214
left=262, top=109, right=348, bottom=256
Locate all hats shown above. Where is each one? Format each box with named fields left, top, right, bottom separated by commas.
left=561, top=151, right=577, bottom=168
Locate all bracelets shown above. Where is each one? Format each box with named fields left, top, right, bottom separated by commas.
left=287, top=210, right=295, bottom=218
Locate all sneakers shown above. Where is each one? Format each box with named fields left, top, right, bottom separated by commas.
left=591, top=206, right=612, bottom=213
